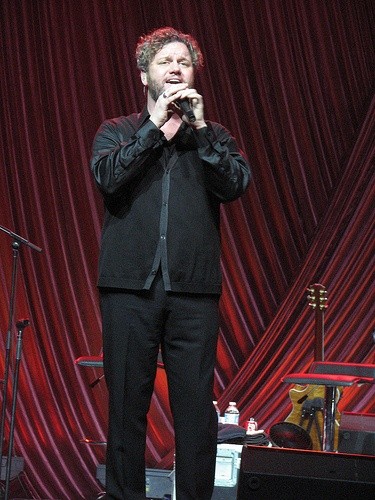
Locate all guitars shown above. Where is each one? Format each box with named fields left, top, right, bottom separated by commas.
left=285, top=283, right=344, bottom=451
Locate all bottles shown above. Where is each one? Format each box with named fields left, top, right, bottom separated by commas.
left=247, top=417, right=258, bottom=431
left=212, top=401, right=220, bottom=421
left=224, top=401, right=239, bottom=426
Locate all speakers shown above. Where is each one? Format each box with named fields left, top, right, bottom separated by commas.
left=236, top=444, right=375, bottom=500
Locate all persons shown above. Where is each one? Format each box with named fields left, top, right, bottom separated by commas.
left=90, top=26, right=251, bottom=500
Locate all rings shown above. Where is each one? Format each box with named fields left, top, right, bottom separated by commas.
left=163, top=93, right=167, bottom=98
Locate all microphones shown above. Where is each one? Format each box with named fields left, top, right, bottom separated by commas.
left=177, top=97, right=196, bottom=122
